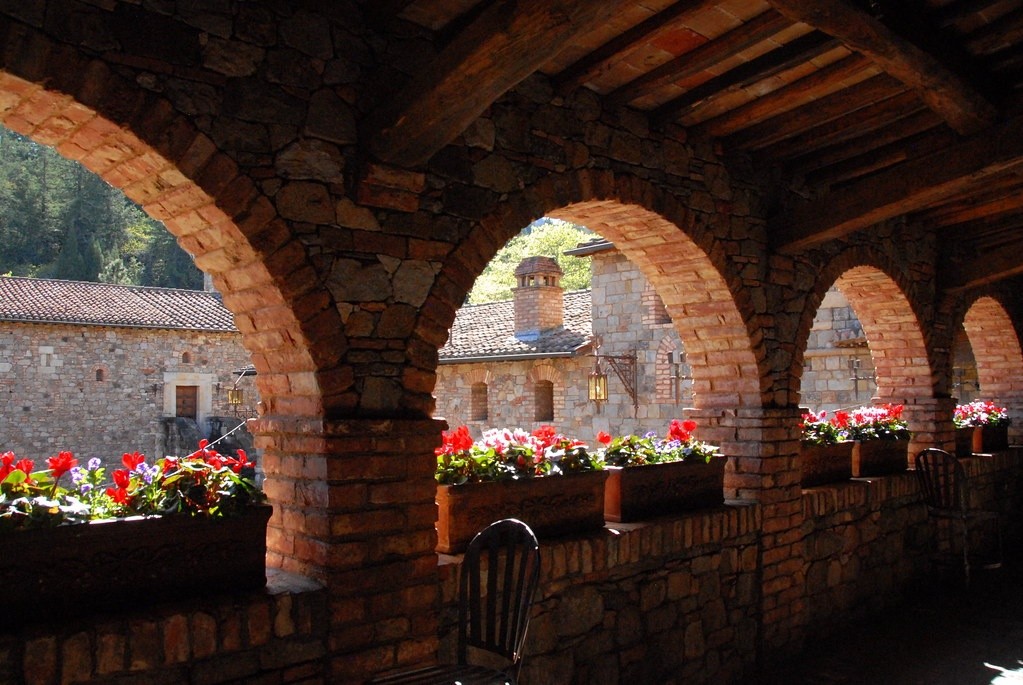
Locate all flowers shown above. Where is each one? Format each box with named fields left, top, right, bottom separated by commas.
left=0, top=439, right=267, bottom=527
left=953, top=400, right=1012, bottom=430
left=598, top=418, right=721, bottom=464
left=434, top=425, right=607, bottom=485
left=799, top=403, right=913, bottom=449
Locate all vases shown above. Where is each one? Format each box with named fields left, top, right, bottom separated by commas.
left=602, top=455, right=729, bottom=524
left=851, top=435, right=909, bottom=478
left=955, top=427, right=976, bottom=457
left=0, top=501, right=274, bottom=636
left=436, top=469, right=610, bottom=553
left=799, top=439, right=855, bottom=488
left=972, top=424, right=1008, bottom=453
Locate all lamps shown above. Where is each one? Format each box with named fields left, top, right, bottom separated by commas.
left=227, top=384, right=244, bottom=417
left=585, top=330, right=638, bottom=420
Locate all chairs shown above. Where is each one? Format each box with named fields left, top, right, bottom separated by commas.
left=915, top=450, right=1007, bottom=627
left=363, top=517, right=542, bottom=685
